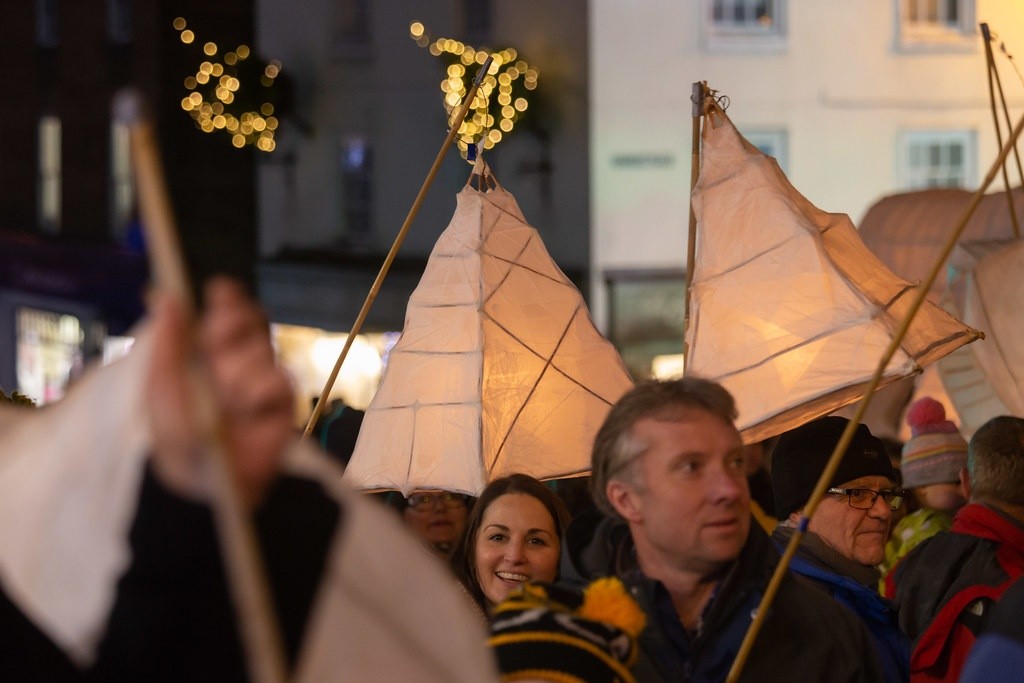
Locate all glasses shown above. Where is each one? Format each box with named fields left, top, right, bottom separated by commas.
left=828, top=488, right=904, bottom=511
left=407, top=490, right=463, bottom=509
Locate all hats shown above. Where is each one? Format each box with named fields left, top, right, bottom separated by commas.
left=770, top=417, right=902, bottom=518
left=901, top=400, right=969, bottom=488
left=485, top=578, right=647, bottom=683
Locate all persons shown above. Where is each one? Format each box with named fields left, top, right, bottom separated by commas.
left=0, top=278, right=1023, bottom=682
